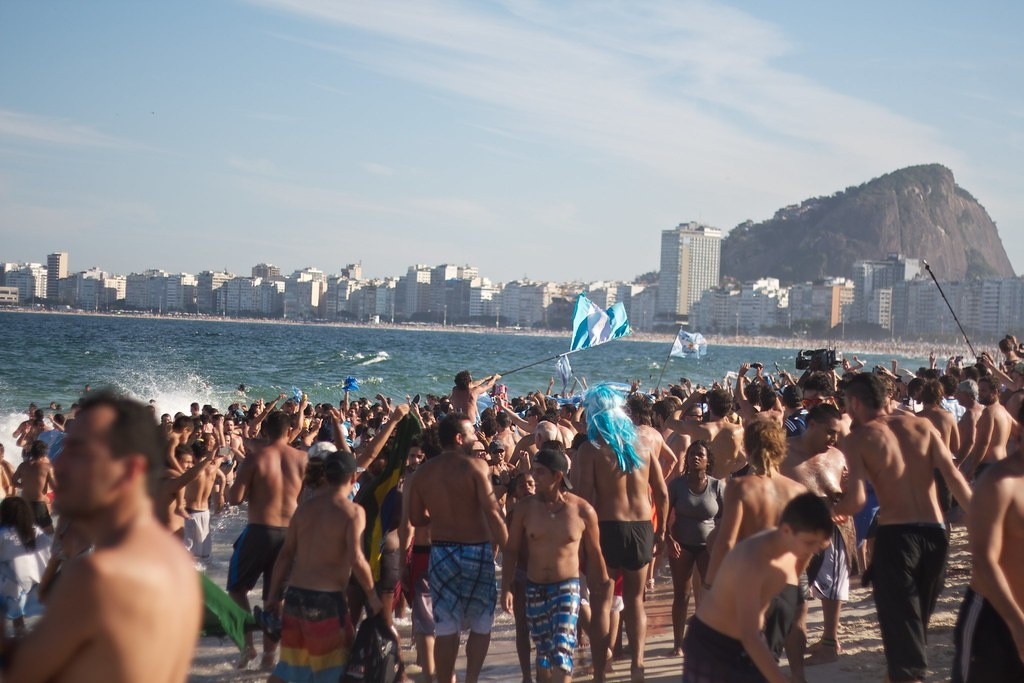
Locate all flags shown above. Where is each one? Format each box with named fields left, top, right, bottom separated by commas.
left=570, top=292, right=630, bottom=352
left=671, top=330, right=707, bottom=360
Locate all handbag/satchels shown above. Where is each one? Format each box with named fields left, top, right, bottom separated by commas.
left=343, top=610, right=406, bottom=683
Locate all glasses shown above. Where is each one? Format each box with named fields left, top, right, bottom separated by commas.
left=492, top=449, right=505, bottom=454
left=688, top=415, right=703, bottom=418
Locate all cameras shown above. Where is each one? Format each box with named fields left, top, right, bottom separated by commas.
left=699, top=394, right=706, bottom=403
left=750, top=363, right=763, bottom=369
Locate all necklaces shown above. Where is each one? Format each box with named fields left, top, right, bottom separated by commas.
left=544, top=499, right=565, bottom=519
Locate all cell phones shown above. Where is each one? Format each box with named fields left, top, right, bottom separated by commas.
left=217, top=447, right=230, bottom=456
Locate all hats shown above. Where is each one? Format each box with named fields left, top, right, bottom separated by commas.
left=532, top=448, right=573, bottom=491
left=307, top=441, right=338, bottom=464
left=907, top=377, right=925, bottom=398
left=784, top=385, right=804, bottom=402
left=325, top=450, right=366, bottom=480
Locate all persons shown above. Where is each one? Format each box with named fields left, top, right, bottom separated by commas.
left=0, top=336, right=1023, bottom=683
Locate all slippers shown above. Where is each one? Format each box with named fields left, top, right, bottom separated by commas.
left=254, top=604, right=283, bottom=643
